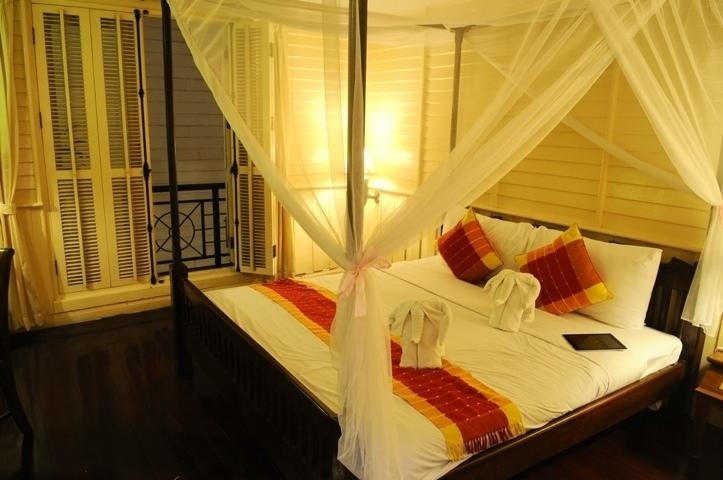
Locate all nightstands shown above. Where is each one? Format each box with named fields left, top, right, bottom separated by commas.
left=688, top=347, right=722, bottom=461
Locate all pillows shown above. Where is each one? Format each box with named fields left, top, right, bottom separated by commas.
left=527, top=226, right=663, bottom=331
left=432, top=205, right=504, bottom=283
left=512, top=222, right=614, bottom=316
left=441, top=200, right=532, bottom=273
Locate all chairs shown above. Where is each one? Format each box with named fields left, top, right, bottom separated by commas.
left=0, top=243, right=35, bottom=440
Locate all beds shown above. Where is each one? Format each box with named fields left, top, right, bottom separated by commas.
left=154, top=2, right=721, bottom=480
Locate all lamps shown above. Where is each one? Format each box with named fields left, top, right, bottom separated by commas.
left=362, top=176, right=382, bottom=210
